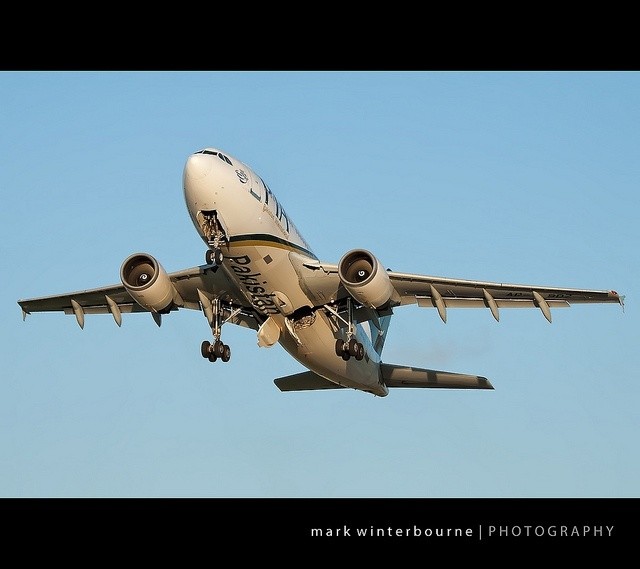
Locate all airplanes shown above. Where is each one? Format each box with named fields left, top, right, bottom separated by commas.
left=16, top=145, right=626, bottom=399
